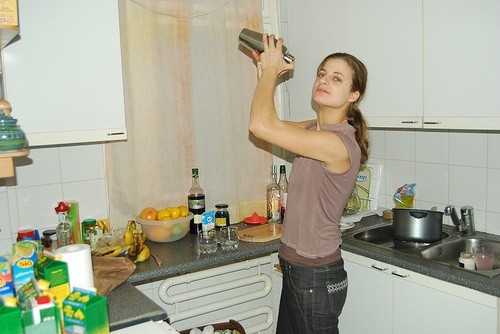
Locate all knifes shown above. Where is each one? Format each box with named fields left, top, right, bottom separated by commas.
left=150, top=249, right=162, bottom=265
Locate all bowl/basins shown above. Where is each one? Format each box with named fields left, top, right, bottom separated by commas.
left=136, top=211, right=194, bottom=243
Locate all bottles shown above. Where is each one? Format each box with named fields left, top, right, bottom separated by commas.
left=266, top=165, right=282, bottom=222
left=459, top=251, right=476, bottom=271
left=83, top=217, right=98, bottom=244
left=0, top=117, right=31, bottom=158
left=54, top=202, right=74, bottom=248
left=215, top=204, right=230, bottom=231
left=238, top=27, right=295, bottom=65
left=278, top=165, right=289, bottom=220
left=188, top=167, right=205, bottom=235
left=17, top=229, right=36, bottom=242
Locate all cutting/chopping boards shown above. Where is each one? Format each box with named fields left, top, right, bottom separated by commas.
left=236, top=222, right=283, bottom=243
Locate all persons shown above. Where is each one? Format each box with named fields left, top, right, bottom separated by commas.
left=248, top=33, right=369, bottom=334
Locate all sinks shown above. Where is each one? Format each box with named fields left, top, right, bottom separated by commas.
left=343, top=219, right=454, bottom=253
left=420, top=235, right=500, bottom=279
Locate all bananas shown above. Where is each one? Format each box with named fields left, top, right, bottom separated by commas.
left=91, top=220, right=150, bottom=263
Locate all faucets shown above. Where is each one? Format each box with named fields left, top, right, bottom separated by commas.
left=444, top=205, right=475, bottom=234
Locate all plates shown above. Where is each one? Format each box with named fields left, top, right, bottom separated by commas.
left=339, top=220, right=355, bottom=230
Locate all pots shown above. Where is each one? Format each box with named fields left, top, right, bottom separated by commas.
left=391, top=207, right=448, bottom=243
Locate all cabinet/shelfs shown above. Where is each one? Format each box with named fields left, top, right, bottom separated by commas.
left=338, top=258, right=500, bottom=334
left=276, top=1, right=500, bottom=130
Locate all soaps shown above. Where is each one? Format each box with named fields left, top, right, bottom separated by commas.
left=383, top=210, right=393, bottom=219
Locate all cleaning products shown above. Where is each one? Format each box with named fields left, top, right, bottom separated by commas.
left=347, top=161, right=371, bottom=210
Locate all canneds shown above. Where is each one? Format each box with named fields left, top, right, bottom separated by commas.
left=14, top=229, right=57, bottom=251
left=83, top=219, right=98, bottom=245
left=215, top=204, right=230, bottom=230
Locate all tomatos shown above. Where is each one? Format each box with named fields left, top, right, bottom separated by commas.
left=139, top=204, right=189, bottom=241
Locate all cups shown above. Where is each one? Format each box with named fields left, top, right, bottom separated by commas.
left=196, top=229, right=217, bottom=254
left=473, top=244, right=495, bottom=271
left=220, top=226, right=240, bottom=251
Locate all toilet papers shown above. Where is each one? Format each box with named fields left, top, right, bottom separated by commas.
left=56, top=244, right=94, bottom=292
